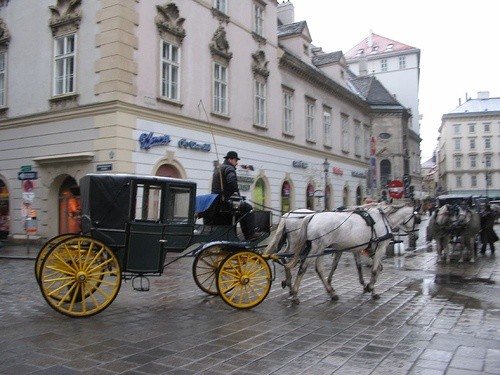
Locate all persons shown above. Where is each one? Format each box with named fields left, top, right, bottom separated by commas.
left=212, top=150, right=265, bottom=243
left=479, top=202, right=499, bottom=254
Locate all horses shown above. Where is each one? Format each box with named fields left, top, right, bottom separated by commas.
left=263, top=196, right=419, bottom=305
left=429, top=203, right=480, bottom=264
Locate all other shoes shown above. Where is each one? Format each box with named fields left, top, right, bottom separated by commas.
left=250, top=231, right=265, bottom=241
left=480, top=249, right=485, bottom=253
left=254, top=227, right=260, bottom=231
left=492, top=249, right=495, bottom=253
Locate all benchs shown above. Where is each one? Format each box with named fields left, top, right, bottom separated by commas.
left=195, top=193, right=240, bottom=216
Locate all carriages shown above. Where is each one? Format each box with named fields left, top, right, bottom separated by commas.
left=35, top=173, right=422, bottom=317
left=426, top=194, right=489, bottom=263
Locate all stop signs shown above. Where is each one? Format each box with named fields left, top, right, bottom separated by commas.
left=389, top=180, right=404, bottom=199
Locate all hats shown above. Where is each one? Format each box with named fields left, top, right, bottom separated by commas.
left=223, top=151, right=240, bottom=160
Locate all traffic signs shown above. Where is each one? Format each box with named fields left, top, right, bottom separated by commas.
left=17, top=171, right=37, bottom=180
